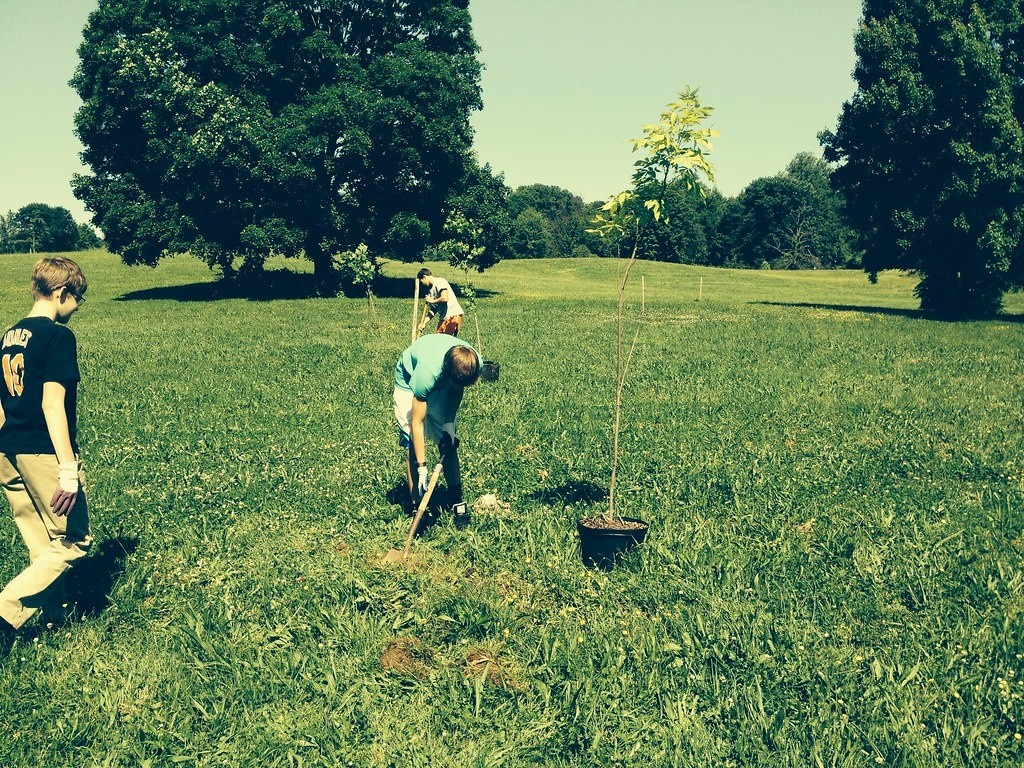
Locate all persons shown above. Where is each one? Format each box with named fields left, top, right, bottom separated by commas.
left=392, top=334, right=483, bottom=538
left=0, top=255, right=94, bottom=640
left=417, top=268, right=465, bottom=337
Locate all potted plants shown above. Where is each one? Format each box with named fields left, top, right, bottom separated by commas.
left=577, top=84, right=722, bottom=568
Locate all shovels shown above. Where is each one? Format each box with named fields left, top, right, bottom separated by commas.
left=381, top=463, right=443, bottom=564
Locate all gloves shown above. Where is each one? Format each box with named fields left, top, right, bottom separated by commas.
left=417, top=466, right=428, bottom=494
left=50, top=453, right=82, bottom=517
left=442, top=423, right=460, bottom=444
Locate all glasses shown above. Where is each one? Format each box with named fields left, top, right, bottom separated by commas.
left=72, top=291, right=85, bottom=306
